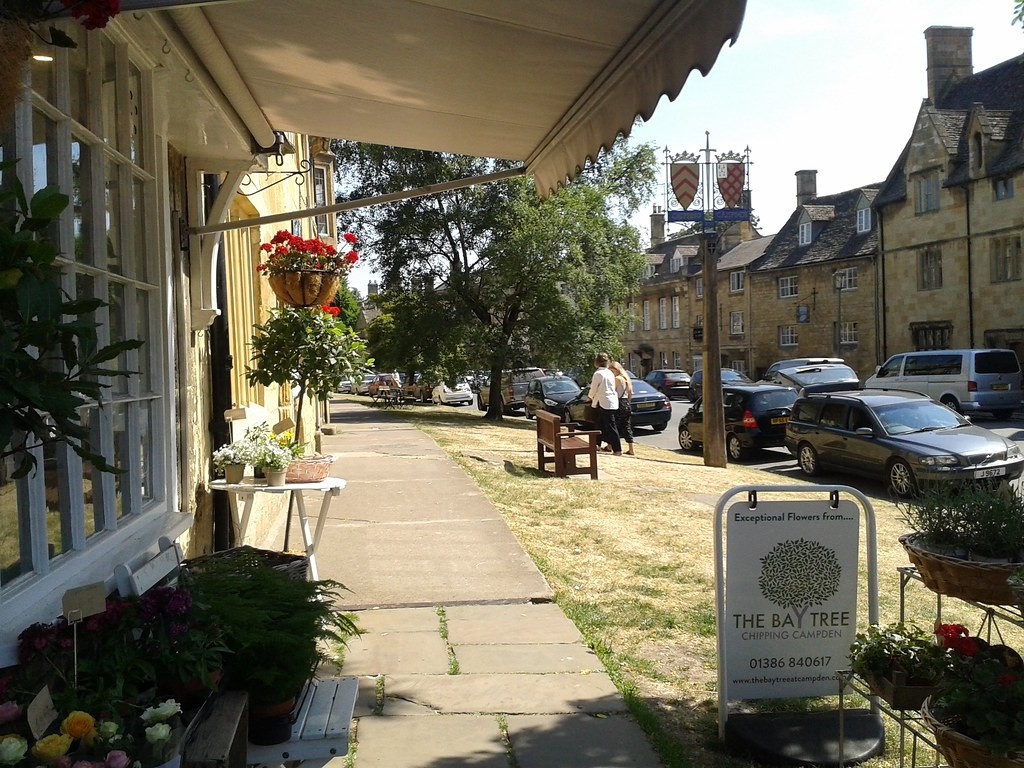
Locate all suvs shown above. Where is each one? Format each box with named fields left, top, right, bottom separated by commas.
left=477, top=367, right=547, bottom=416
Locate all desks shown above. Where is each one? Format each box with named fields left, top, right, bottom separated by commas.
left=210, top=475, right=347, bottom=630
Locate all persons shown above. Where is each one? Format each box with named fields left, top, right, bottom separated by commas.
left=367, top=381, right=398, bottom=397
left=587, top=353, right=622, bottom=456
left=392, top=369, right=399, bottom=384
left=601, top=360, right=634, bottom=455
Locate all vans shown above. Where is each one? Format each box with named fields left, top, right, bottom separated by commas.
left=861, top=348, right=1024, bottom=421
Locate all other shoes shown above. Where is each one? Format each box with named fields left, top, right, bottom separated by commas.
left=623, top=450, right=635, bottom=455
left=600, top=446, right=613, bottom=452
left=607, top=451, right=622, bottom=456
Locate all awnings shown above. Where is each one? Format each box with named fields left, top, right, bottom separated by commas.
left=0, top=0, right=747, bottom=238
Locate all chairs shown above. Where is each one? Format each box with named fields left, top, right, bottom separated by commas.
left=114, top=536, right=359, bottom=768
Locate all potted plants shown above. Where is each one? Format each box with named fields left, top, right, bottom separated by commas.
left=190, top=547, right=370, bottom=746
left=845, top=617, right=953, bottom=711
left=887, top=456, right=1024, bottom=606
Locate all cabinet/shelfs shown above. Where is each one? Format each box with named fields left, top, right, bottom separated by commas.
left=836, top=568, right=1023, bottom=768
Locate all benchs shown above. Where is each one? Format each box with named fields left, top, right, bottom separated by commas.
left=370, top=388, right=417, bottom=410
left=536, top=410, right=602, bottom=481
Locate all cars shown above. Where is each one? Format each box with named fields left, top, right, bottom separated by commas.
left=785, top=387, right=1024, bottom=501
left=432, top=376, right=474, bottom=406
left=563, top=369, right=672, bottom=431
left=762, top=357, right=861, bottom=391
left=687, top=368, right=756, bottom=404
left=524, top=375, right=583, bottom=426
left=678, top=384, right=799, bottom=464
left=333, top=371, right=484, bottom=404
left=643, top=368, right=692, bottom=400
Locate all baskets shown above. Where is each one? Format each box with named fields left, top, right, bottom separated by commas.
left=287, top=454, right=332, bottom=483
left=920, top=693, right=1024, bottom=768
left=178, top=546, right=308, bottom=598
left=900, top=529, right=1023, bottom=605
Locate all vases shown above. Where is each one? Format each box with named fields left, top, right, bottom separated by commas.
left=254, top=466, right=265, bottom=478
left=264, top=467, right=287, bottom=486
left=225, top=464, right=245, bottom=484
left=191, top=669, right=221, bottom=691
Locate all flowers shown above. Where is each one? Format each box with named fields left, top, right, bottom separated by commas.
left=256, top=230, right=359, bottom=277
left=932, top=622, right=1024, bottom=758
left=212, top=421, right=310, bottom=476
left=0, top=0, right=123, bottom=48
left=0, top=586, right=236, bottom=768
left=322, top=297, right=340, bottom=317
